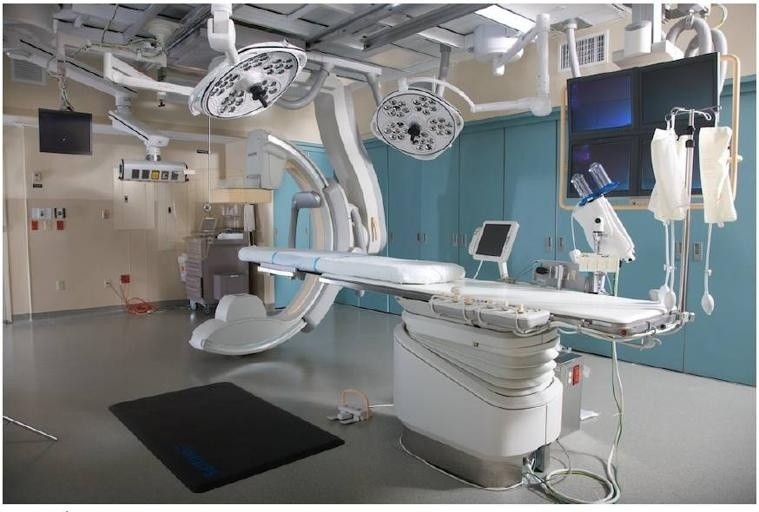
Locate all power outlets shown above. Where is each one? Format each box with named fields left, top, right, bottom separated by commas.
left=104, top=280, right=111, bottom=288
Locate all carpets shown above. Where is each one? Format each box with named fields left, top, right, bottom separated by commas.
left=107, top=381, right=346, bottom=493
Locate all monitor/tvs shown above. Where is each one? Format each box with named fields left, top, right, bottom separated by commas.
left=566, top=66, right=638, bottom=136
left=36, top=106, right=93, bottom=156
left=474, top=220, right=519, bottom=261
left=639, top=112, right=718, bottom=196
left=567, top=130, right=639, bottom=198
left=638, top=50, right=721, bottom=124
